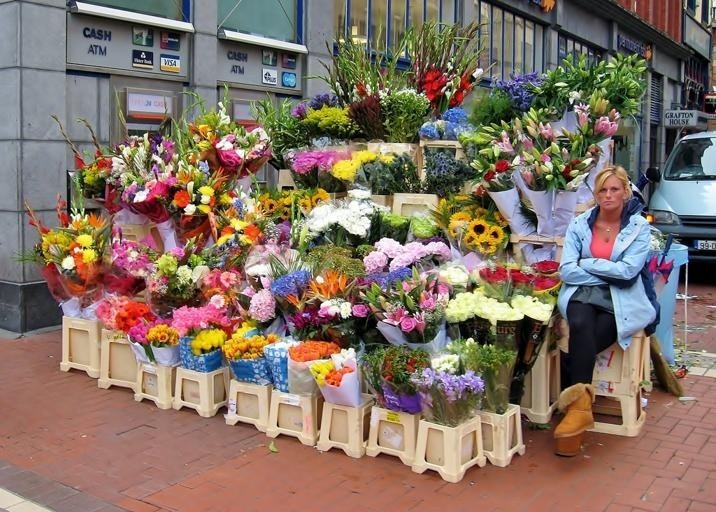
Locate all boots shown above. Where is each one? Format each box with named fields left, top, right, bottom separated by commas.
left=553, top=383, right=595, bottom=458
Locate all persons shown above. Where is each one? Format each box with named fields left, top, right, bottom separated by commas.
left=548, top=163, right=657, bottom=458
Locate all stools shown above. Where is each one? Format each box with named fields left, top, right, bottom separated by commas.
left=560, top=318, right=651, bottom=437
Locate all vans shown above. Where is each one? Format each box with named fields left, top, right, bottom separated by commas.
left=645, top=131, right=716, bottom=263
